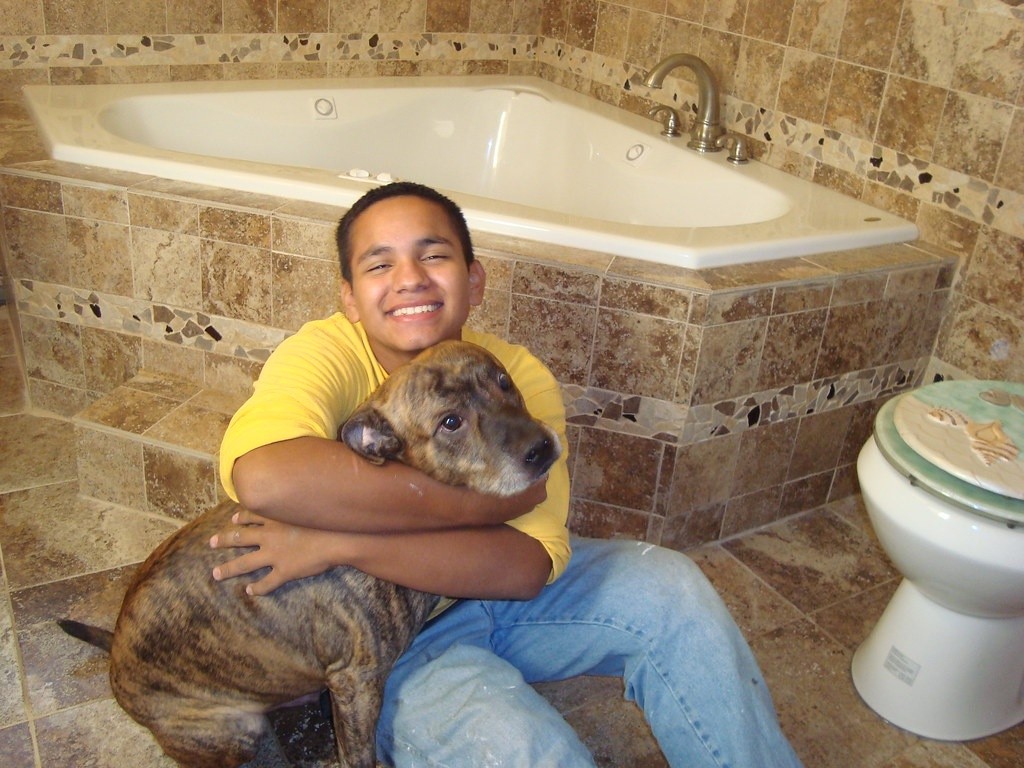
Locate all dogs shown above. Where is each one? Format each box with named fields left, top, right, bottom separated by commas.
left=54, top=339, right=562, bottom=768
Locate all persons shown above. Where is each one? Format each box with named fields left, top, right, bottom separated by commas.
left=209, top=182, right=805, bottom=768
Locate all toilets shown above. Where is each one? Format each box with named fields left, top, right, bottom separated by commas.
left=850, top=377, right=1023, bottom=742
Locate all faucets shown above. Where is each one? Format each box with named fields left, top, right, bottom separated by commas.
left=644, top=53, right=725, bottom=153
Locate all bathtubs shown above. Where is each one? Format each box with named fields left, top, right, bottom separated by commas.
left=0, top=76, right=958, bottom=554
